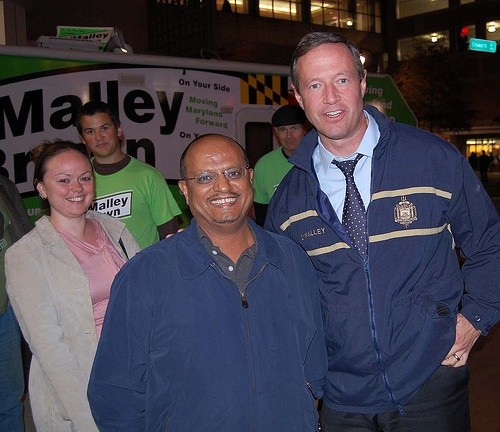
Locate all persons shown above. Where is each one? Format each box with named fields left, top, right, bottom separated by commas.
left=0, top=174, right=37, bottom=432
left=264, top=31, right=500, bottom=432
left=87, top=133, right=327, bottom=432
left=252, top=105, right=308, bottom=229
left=4, top=139, right=188, bottom=432
left=467, top=150, right=495, bottom=182
left=76, top=101, right=182, bottom=250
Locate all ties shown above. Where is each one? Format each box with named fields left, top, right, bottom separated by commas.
left=332, top=154, right=371, bottom=258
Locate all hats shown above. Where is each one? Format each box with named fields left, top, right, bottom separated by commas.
left=271, top=103, right=308, bottom=127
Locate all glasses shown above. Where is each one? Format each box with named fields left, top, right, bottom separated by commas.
left=185, top=165, right=251, bottom=186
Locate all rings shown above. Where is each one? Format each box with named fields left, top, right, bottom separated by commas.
left=453, top=353, right=460, bottom=361
